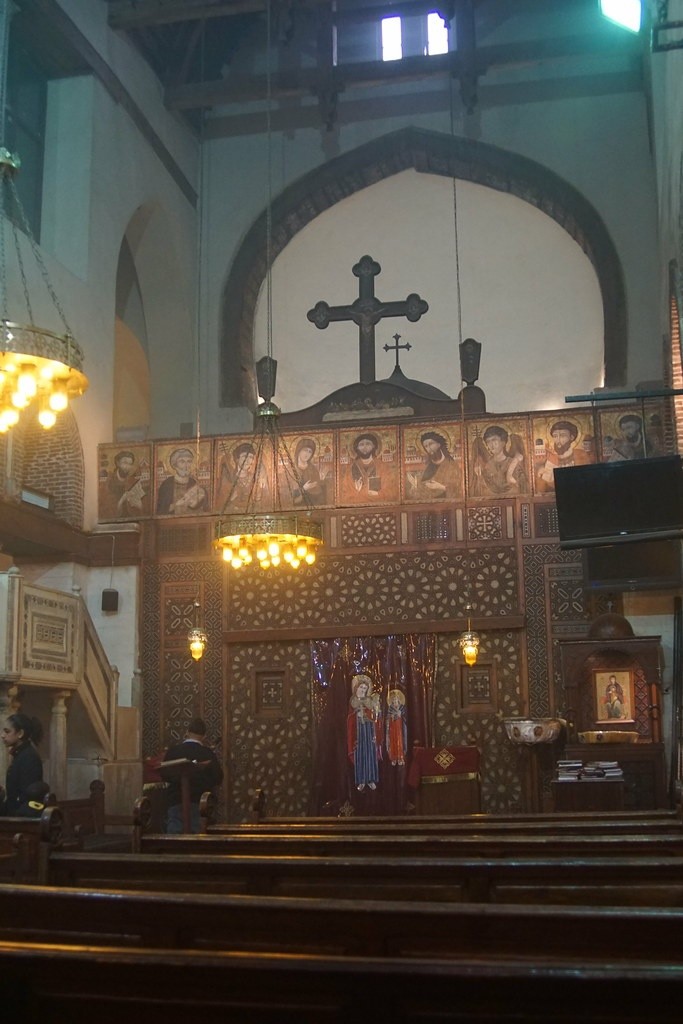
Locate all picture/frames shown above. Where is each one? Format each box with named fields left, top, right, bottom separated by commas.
left=97, top=401, right=663, bottom=524
left=592, top=668, right=636, bottom=724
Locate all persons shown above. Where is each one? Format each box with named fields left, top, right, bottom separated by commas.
left=164, top=718, right=223, bottom=834
left=0, top=714, right=44, bottom=817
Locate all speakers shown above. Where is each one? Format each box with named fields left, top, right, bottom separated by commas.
left=102, top=589, right=119, bottom=612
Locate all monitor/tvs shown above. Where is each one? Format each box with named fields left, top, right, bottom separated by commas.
left=553, top=453, right=683, bottom=551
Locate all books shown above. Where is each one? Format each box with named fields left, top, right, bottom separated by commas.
left=556, top=760, right=623, bottom=781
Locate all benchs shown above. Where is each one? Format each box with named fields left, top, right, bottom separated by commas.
left=0, top=789, right=683, bottom=1024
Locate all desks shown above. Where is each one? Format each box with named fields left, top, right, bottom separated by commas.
left=550, top=779, right=625, bottom=812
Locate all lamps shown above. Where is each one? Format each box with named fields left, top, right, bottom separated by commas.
left=212, top=0, right=323, bottom=569
left=189, top=603, right=207, bottom=661
left=458, top=605, right=482, bottom=666
left=0, top=127, right=89, bottom=433
